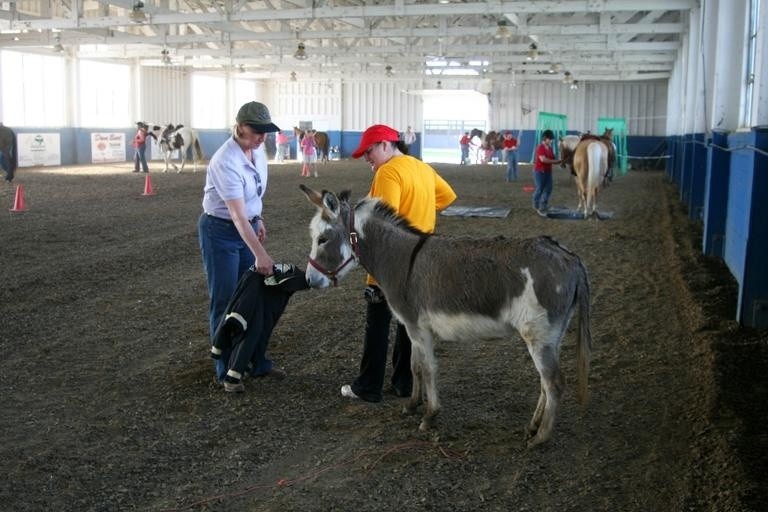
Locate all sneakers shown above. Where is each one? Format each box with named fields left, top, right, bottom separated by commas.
left=222, top=379, right=246, bottom=392
left=341, top=385, right=361, bottom=399
left=244, top=366, right=288, bottom=383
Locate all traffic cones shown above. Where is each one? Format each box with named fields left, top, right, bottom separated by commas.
left=139, top=173, right=156, bottom=196
left=8, top=184, right=28, bottom=212
left=301, top=163, right=309, bottom=177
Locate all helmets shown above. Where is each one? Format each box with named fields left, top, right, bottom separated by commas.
left=543, top=130, right=555, bottom=139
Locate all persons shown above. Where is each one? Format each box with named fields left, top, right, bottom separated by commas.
left=402, top=126, right=416, bottom=155
left=198, top=102, right=287, bottom=393
left=459, top=132, right=477, bottom=165
left=275, top=127, right=319, bottom=178
left=502, top=130, right=519, bottom=182
left=341, top=124, right=457, bottom=402
left=131, top=122, right=149, bottom=173
left=531, top=129, right=562, bottom=217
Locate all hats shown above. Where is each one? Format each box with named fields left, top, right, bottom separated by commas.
left=236, top=102, right=281, bottom=132
left=351, top=125, right=399, bottom=158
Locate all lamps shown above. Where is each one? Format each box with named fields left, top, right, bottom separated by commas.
left=238, top=64, right=446, bottom=92
left=125, top=1, right=150, bottom=24
left=48, top=30, right=66, bottom=56
left=493, top=16, right=578, bottom=91
left=291, top=43, right=310, bottom=59
left=159, top=47, right=172, bottom=65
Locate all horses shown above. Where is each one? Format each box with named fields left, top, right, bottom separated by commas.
left=134, top=121, right=202, bottom=175
left=0, top=124, right=17, bottom=182
left=470, top=128, right=504, bottom=164
left=558, top=126, right=617, bottom=220
left=293, top=126, right=329, bottom=162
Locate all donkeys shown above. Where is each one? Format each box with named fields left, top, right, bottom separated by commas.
left=297, top=183, right=591, bottom=450
